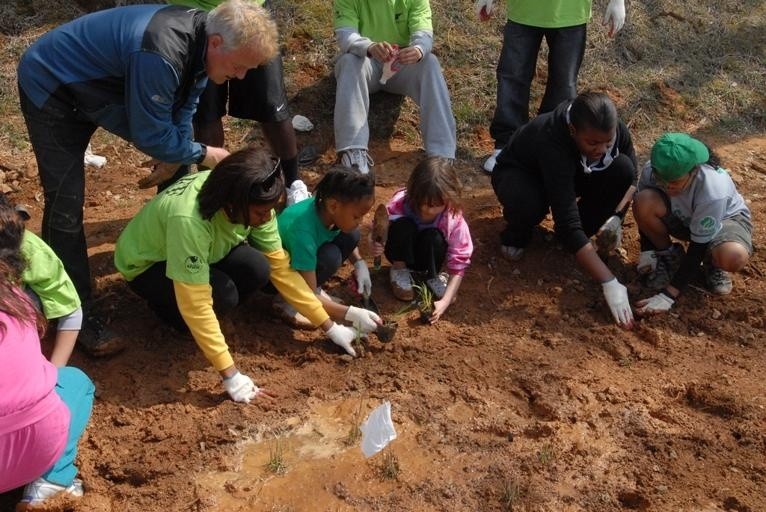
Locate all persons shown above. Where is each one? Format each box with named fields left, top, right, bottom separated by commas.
left=632, top=131, right=753, bottom=314
left=0, top=205, right=85, bottom=367
left=276, top=168, right=385, bottom=333
left=0, top=249, right=97, bottom=507
left=491, top=89, right=638, bottom=326
left=332, top=1, right=457, bottom=173
left=367, top=157, right=475, bottom=325
left=480, top=0, right=626, bottom=173
left=155, top=0, right=307, bottom=206
left=16, top=0, right=279, bottom=358
left=114, top=148, right=371, bottom=404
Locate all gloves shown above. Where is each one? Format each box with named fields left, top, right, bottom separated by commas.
left=598, top=215, right=623, bottom=248
left=637, top=251, right=657, bottom=273
left=291, top=114, right=314, bottom=132
left=325, top=320, right=370, bottom=357
left=600, top=276, right=636, bottom=330
left=380, top=44, right=402, bottom=86
left=344, top=305, right=383, bottom=333
left=603, top=1, right=626, bottom=38
left=475, top=0, right=492, bottom=20
left=637, top=294, right=675, bottom=315
left=354, top=259, right=372, bottom=297
left=222, top=371, right=264, bottom=404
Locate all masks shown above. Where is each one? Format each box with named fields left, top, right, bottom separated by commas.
left=587, top=151, right=614, bottom=172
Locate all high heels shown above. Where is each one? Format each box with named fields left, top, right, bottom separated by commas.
left=147, top=305, right=195, bottom=343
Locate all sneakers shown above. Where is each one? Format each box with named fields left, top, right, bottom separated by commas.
left=426, top=272, right=456, bottom=305
left=271, top=293, right=315, bottom=329
left=501, top=246, right=523, bottom=259
left=483, top=149, right=502, bottom=173
left=389, top=264, right=415, bottom=301
left=17, top=477, right=84, bottom=508
left=702, top=262, right=734, bottom=295
left=245, top=290, right=275, bottom=308
left=317, top=286, right=344, bottom=305
left=646, top=242, right=687, bottom=291
left=286, top=180, right=312, bottom=207
left=77, top=316, right=124, bottom=356
left=341, top=149, right=374, bottom=174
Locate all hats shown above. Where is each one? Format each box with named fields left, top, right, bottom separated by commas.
left=651, top=132, right=710, bottom=181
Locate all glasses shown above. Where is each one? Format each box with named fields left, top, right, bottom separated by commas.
left=254, top=156, right=282, bottom=192
left=649, top=169, right=692, bottom=192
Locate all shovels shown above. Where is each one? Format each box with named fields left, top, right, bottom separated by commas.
left=370, top=203, right=390, bottom=271
